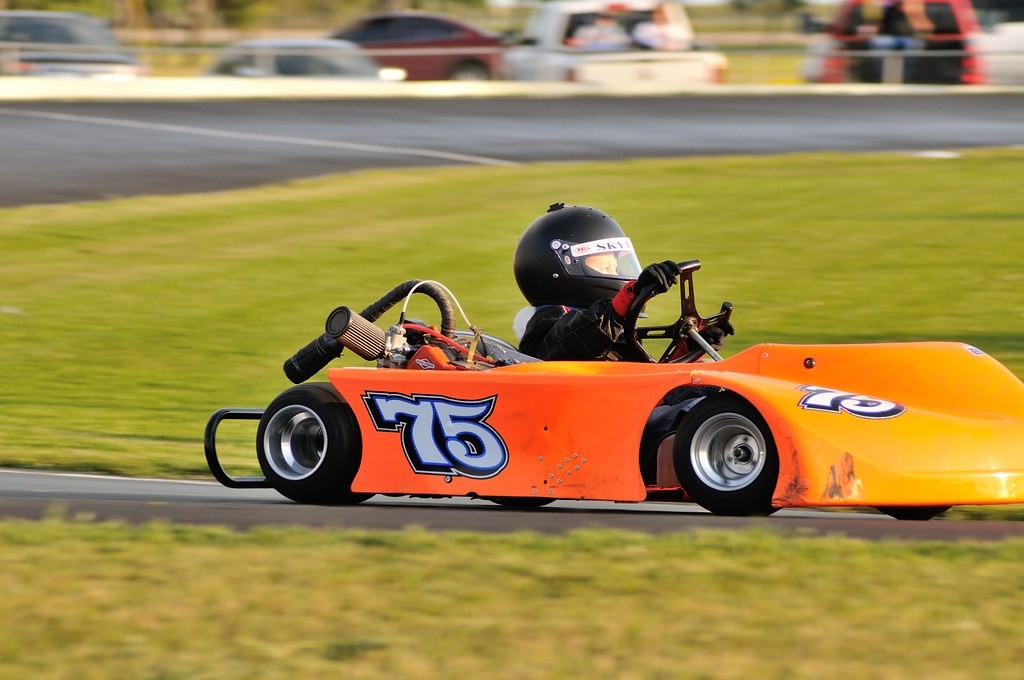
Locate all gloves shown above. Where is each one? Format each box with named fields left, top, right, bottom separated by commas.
left=685, top=322, right=734, bottom=351
left=634, top=260, right=682, bottom=298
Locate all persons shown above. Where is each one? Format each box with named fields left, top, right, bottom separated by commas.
left=830, top=0, right=987, bottom=84
left=565, top=0, right=693, bottom=51
left=514, top=203, right=723, bottom=363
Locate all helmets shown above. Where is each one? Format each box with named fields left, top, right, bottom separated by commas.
left=513, top=203, right=648, bottom=318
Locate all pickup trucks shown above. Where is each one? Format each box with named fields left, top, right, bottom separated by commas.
left=501, top=1, right=735, bottom=96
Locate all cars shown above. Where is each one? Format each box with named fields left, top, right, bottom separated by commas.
left=329, top=9, right=512, bottom=82
left=798, top=0, right=1024, bottom=84
left=199, top=39, right=384, bottom=80
left=0, top=8, right=145, bottom=73
